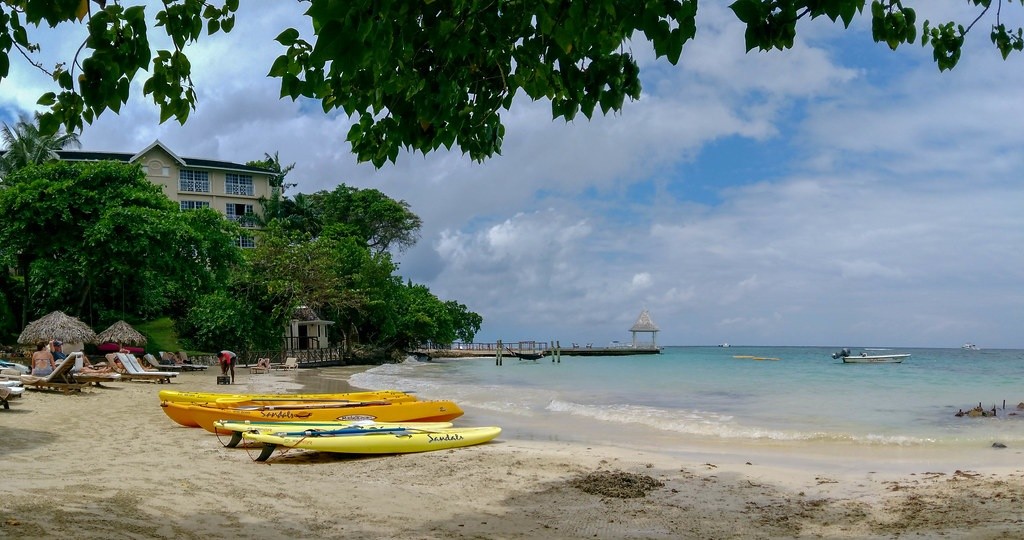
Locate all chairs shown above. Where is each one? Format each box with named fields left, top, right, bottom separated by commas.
left=0, top=351, right=208, bottom=410
left=276, top=358, right=298, bottom=371
left=250, top=358, right=270, bottom=374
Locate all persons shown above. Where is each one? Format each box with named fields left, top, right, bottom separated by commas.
left=217, top=350, right=236, bottom=384
left=80, top=349, right=103, bottom=388
left=32, top=342, right=57, bottom=390
left=49, top=341, right=111, bottom=374
left=113, top=349, right=151, bottom=372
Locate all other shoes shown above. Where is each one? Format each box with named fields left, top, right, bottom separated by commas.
left=231, top=382, right=234, bottom=384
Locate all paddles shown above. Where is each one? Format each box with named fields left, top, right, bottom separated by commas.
left=220, top=419, right=376, bottom=427
left=279, top=428, right=444, bottom=436
left=229, top=402, right=391, bottom=411
left=216, top=397, right=385, bottom=403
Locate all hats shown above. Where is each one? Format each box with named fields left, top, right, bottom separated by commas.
left=54, top=341, right=63, bottom=345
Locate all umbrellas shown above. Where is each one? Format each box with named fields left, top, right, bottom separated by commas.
left=18, top=311, right=96, bottom=343
left=96, top=320, right=147, bottom=349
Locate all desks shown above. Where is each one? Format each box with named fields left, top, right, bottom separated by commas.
left=93, top=364, right=107, bottom=371
left=158, top=359, right=172, bottom=372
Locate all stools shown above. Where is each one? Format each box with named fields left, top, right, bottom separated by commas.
left=216, top=376, right=229, bottom=385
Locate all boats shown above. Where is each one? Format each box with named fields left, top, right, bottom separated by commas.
left=719, top=344, right=731, bottom=347
left=962, top=343, right=979, bottom=351
left=157, top=390, right=504, bottom=454
left=832, top=347, right=912, bottom=364
